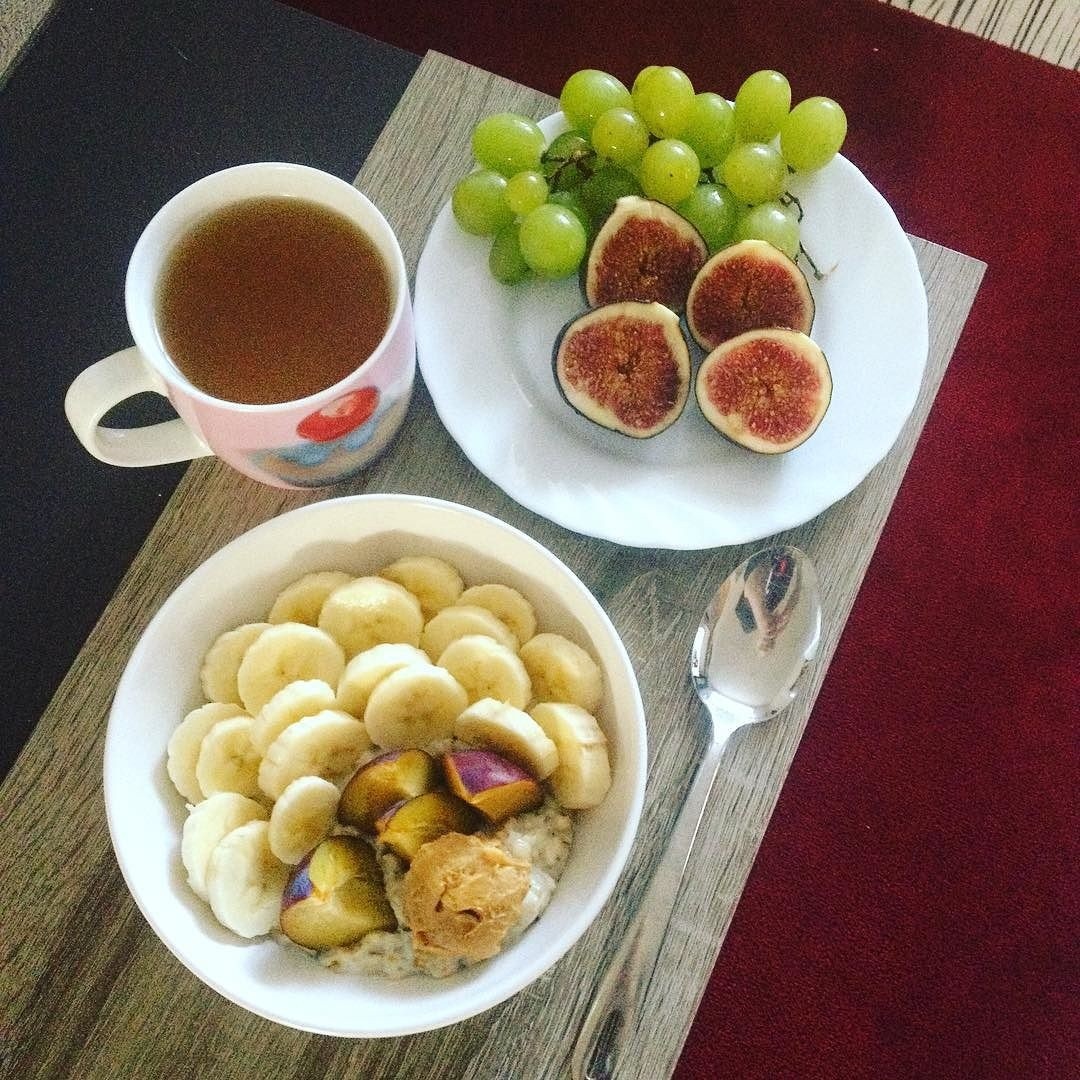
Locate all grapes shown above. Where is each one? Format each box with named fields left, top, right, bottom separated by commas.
left=449, top=64, right=848, bottom=282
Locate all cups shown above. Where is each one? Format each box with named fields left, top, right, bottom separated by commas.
left=64, top=161, right=418, bottom=491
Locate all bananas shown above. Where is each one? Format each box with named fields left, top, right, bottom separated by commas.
left=167, top=557, right=612, bottom=940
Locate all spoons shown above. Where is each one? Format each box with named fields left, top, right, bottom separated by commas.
left=570, top=546, right=823, bottom=1080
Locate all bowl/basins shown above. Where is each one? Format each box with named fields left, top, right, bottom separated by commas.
left=102, top=494, right=649, bottom=1041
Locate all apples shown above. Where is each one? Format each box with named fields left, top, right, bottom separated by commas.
left=338, top=748, right=438, bottom=832
left=442, top=750, right=542, bottom=826
left=375, top=791, right=479, bottom=866
left=279, top=834, right=395, bottom=952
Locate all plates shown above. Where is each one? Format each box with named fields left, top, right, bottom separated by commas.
left=412, top=102, right=927, bottom=549
